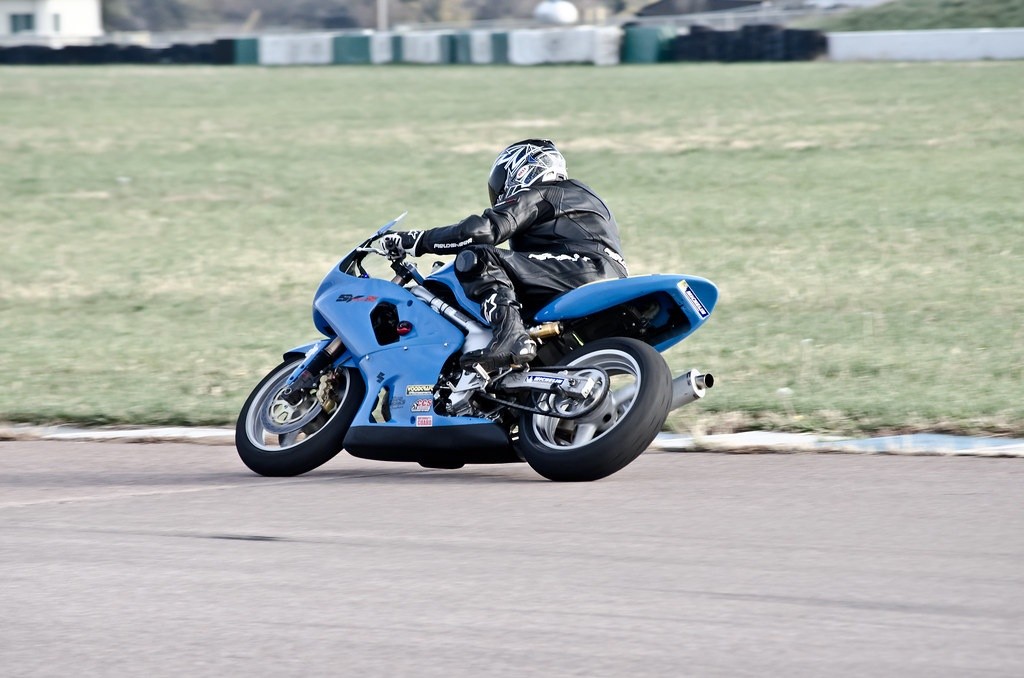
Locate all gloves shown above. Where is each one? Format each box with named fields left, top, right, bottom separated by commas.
left=377, top=228, right=426, bottom=258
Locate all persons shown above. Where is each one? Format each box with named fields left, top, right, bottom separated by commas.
left=377, top=138, right=630, bottom=374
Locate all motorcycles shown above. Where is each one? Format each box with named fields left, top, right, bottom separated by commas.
left=235, top=211, right=718, bottom=482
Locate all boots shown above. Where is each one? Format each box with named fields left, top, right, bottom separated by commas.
left=459, top=289, right=538, bottom=372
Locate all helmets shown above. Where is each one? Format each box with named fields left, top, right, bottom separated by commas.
left=488, top=138, right=568, bottom=210
